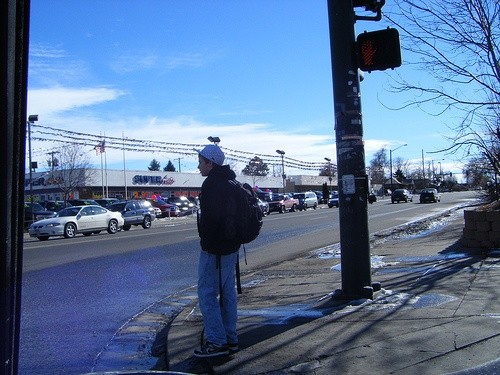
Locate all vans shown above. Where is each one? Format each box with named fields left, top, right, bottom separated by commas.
left=295, top=192, right=319, bottom=211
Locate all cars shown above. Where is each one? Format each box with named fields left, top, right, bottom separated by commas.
left=29, top=206, right=125, bottom=242
left=256, top=198, right=270, bottom=217
left=71, top=199, right=102, bottom=209
left=267, top=193, right=300, bottom=213
left=109, top=199, right=156, bottom=231
left=42, top=200, right=73, bottom=213
left=327, top=190, right=340, bottom=208
left=420, top=188, right=441, bottom=204
left=24, top=201, right=57, bottom=226
left=151, top=195, right=201, bottom=219
left=96, top=198, right=120, bottom=207
left=390, top=189, right=414, bottom=203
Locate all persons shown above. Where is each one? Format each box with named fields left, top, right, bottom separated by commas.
left=152, top=192, right=161, bottom=200
left=193, top=144, right=241, bottom=358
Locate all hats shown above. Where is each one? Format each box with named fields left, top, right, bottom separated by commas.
left=193, top=144, right=225, bottom=166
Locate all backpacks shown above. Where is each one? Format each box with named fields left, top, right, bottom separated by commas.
left=218, top=176, right=265, bottom=244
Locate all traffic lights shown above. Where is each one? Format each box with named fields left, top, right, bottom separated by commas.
left=357, top=27, right=403, bottom=73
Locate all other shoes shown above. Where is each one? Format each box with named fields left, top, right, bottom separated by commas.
left=228, top=343, right=239, bottom=351
left=193, top=342, right=230, bottom=356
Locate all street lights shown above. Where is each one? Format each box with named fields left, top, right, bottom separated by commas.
left=389, top=144, right=408, bottom=193
left=28, top=115, right=39, bottom=225
left=324, top=157, right=332, bottom=191
left=276, top=149, right=285, bottom=193
left=432, top=158, right=445, bottom=183
